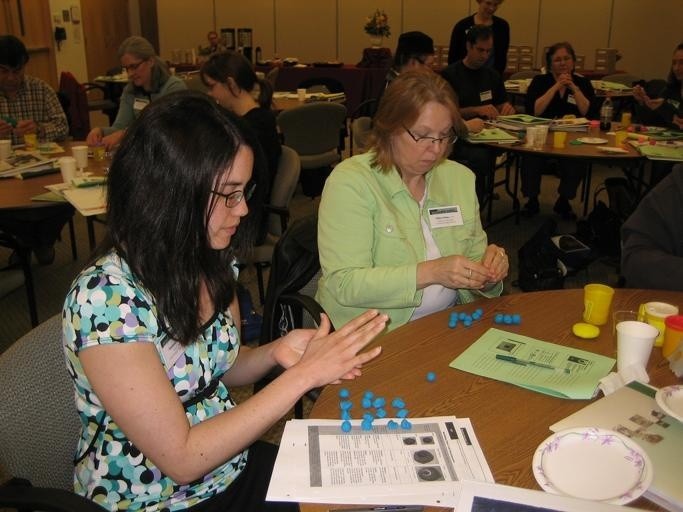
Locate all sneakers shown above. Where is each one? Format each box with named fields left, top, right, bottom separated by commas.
left=524, top=199, right=540, bottom=215
left=552, top=197, right=578, bottom=222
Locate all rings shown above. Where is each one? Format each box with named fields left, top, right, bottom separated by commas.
left=467, top=269, right=473, bottom=279
left=498, top=250, right=504, bottom=257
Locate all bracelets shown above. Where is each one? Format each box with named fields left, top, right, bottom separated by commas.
left=570, top=87, right=580, bottom=94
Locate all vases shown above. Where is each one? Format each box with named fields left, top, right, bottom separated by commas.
left=368, top=35, right=384, bottom=49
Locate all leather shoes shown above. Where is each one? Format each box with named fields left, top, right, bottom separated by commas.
left=33, top=242, right=56, bottom=265
left=9, top=244, right=33, bottom=267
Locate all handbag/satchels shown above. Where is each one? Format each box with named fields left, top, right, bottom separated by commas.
left=563, top=228, right=604, bottom=265
left=590, top=200, right=624, bottom=259
left=58, top=71, right=110, bottom=140
left=235, top=282, right=263, bottom=346
left=517, top=250, right=564, bottom=292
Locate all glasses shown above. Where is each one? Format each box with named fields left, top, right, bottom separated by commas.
left=416, top=57, right=433, bottom=68
left=120, top=60, right=146, bottom=69
left=400, top=121, right=458, bottom=145
left=210, top=184, right=256, bottom=208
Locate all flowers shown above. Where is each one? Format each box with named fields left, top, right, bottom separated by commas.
left=363, top=7, right=392, bottom=39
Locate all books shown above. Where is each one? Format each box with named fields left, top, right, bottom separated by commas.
left=502, top=77, right=534, bottom=91
left=589, top=79, right=634, bottom=96
left=1, top=140, right=107, bottom=218
left=464, top=110, right=683, bottom=163
left=551, top=378, right=682, bottom=512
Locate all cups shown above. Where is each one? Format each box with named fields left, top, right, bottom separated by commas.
left=525, top=125, right=549, bottom=150
left=71, top=145, right=89, bottom=168
left=621, top=112, right=631, bottom=127
left=297, top=88, right=306, bottom=102
left=614, top=320, right=659, bottom=376
left=553, top=131, right=567, bottom=149
left=0, top=139, right=11, bottom=159
left=641, top=300, right=678, bottom=347
left=583, top=283, right=614, bottom=329
left=24, top=134, right=37, bottom=151
left=93, top=145, right=105, bottom=161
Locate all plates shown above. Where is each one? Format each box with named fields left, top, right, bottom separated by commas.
left=533, top=423, right=654, bottom=507
left=576, top=136, right=608, bottom=145
left=655, top=382, right=683, bottom=427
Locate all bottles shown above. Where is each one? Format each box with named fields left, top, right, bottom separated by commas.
left=600, top=93, right=613, bottom=132
left=58, top=156, right=76, bottom=183
left=662, top=315, right=683, bottom=360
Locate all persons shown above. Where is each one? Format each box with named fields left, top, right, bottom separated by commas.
left=618, top=160, right=682, bottom=292
left=198, top=49, right=285, bottom=250
left=311, top=55, right=512, bottom=336
left=435, top=23, right=517, bottom=214
left=0, top=34, right=73, bottom=273
left=368, top=30, right=435, bottom=130
left=517, top=38, right=602, bottom=221
left=630, top=43, right=683, bottom=190
left=446, top=0, right=511, bottom=75
left=195, top=30, right=228, bottom=57
left=59, top=88, right=391, bottom=512
left=86, top=36, right=189, bottom=158
